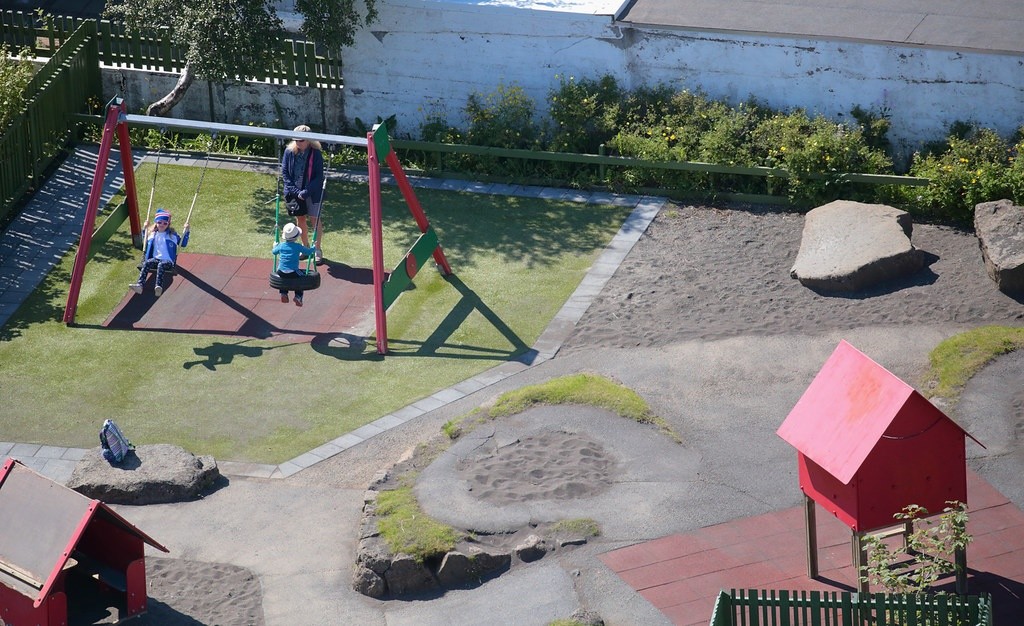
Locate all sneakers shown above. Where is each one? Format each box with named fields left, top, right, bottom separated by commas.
left=299, top=243, right=310, bottom=259
left=293, top=295, right=303, bottom=307
left=312, top=249, right=322, bottom=264
left=281, top=293, right=289, bottom=303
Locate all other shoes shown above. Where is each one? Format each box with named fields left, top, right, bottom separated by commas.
left=129, top=283, right=143, bottom=294
left=154, top=285, right=163, bottom=296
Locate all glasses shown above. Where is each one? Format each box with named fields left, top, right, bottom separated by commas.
left=295, top=139, right=305, bottom=142
left=157, top=221, right=168, bottom=225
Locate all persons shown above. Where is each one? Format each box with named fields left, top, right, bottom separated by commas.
left=272, top=222, right=321, bottom=307
left=128, top=208, right=190, bottom=297
left=281, top=124, right=326, bottom=266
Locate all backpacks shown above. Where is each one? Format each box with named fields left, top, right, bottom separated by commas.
left=100, top=419, right=136, bottom=463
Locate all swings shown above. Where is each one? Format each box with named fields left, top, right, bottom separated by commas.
left=136, top=128, right=216, bottom=276
left=268, top=138, right=335, bottom=291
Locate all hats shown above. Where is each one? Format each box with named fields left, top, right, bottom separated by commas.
left=282, top=222, right=303, bottom=240
left=292, top=124, right=311, bottom=141
left=155, top=208, right=170, bottom=222
